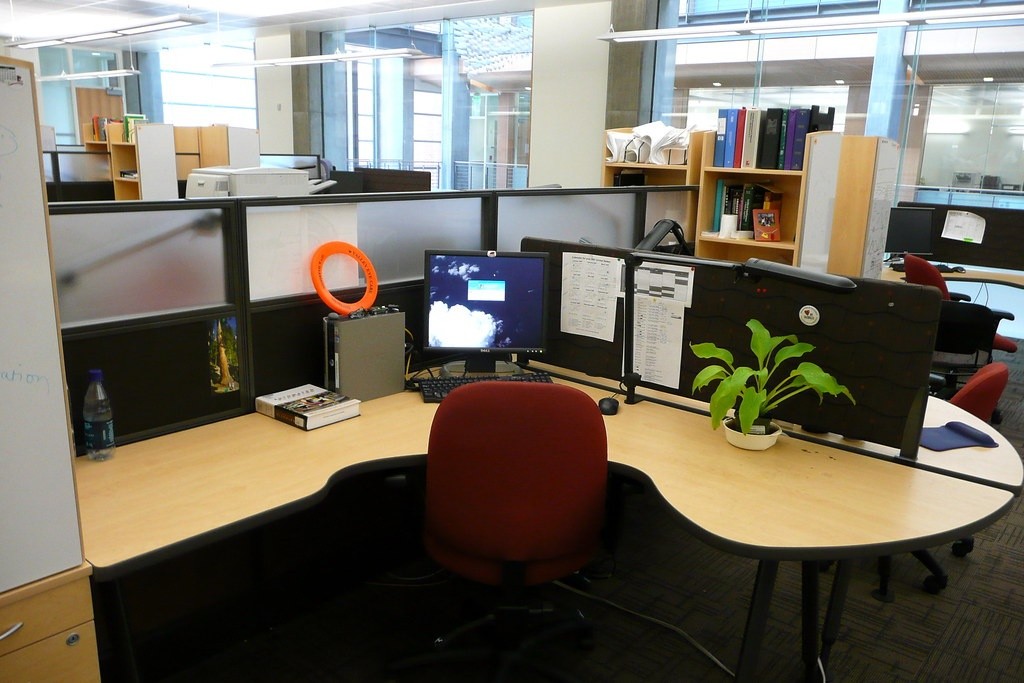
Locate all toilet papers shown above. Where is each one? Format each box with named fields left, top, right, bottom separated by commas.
left=718, top=213, right=738, bottom=238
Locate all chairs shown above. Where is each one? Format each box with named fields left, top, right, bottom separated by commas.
left=383, top=388, right=607, bottom=683
left=901, top=251, right=1017, bottom=424
left=823, top=362, right=1010, bottom=601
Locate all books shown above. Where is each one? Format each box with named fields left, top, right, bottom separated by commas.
left=120, top=170, right=137, bottom=179
left=713, top=104, right=836, bottom=171
left=712, top=177, right=785, bottom=242
left=91, top=116, right=124, bottom=142
left=254, top=384, right=362, bottom=432
left=123, top=114, right=149, bottom=143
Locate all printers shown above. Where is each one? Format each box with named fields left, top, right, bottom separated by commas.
left=186, top=165, right=338, bottom=201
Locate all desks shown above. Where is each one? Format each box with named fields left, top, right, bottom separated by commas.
left=74, top=351, right=1023, bottom=683
left=877, top=264, right=1024, bottom=291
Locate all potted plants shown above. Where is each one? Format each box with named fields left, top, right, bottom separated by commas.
left=689, top=320, right=856, bottom=449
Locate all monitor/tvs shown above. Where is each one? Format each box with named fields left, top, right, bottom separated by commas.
left=883, top=208, right=933, bottom=265
left=424, top=250, right=550, bottom=378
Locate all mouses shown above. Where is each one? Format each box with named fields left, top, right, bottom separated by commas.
left=952, top=267, right=965, bottom=273
left=599, top=398, right=619, bottom=416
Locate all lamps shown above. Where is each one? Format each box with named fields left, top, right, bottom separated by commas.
left=34, top=35, right=141, bottom=82
left=622, top=219, right=858, bottom=406
left=210, top=1, right=427, bottom=67
left=597, top=0, right=1024, bottom=46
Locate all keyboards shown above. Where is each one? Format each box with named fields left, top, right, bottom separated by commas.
left=420, top=373, right=554, bottom=404
left=891, top=264, right=955, bottom=272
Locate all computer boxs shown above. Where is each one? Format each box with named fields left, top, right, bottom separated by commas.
left=322, top=309, right=405, bottom=401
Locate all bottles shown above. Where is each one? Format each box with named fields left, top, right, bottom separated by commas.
left=82, top=369, right=115, bottom=462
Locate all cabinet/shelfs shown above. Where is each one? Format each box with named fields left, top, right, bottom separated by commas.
left=601, top=125, right=903, bottom=278
left=0, top=562, right=102, bottom=683
left=83, top=121, right=178, bottom=201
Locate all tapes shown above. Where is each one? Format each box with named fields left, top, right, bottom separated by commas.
left=731, top=230, right=754, bottom=239
